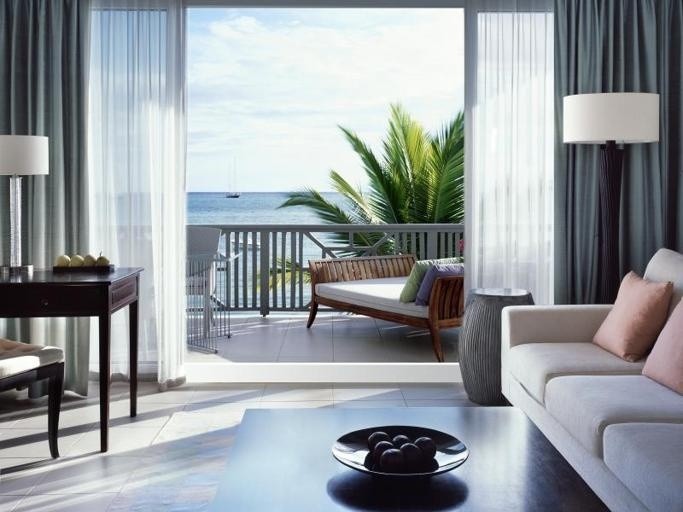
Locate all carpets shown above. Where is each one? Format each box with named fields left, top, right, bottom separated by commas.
left=100, top=408, right=247, bottom=512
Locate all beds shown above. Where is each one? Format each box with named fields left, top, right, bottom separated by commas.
left=300, top=249, right=466, bottom=364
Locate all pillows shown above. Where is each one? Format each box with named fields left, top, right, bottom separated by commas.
left=637, top=295, right=682, bottom=399
left=397, top=256, right=463, bottom=303
left=587, top=268, right=674, bottom=363
left=412, top=262, right=464, bottom=306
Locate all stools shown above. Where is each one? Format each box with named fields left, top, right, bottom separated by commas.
left=454, top=287, right=540, bottom=405
left=0, top=336, right=64, bottom=462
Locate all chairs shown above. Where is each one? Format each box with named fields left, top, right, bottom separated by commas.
left=117, top=223, right=242, bottom=339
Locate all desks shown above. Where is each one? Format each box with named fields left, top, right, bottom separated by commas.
left=0, top=266, right=147, bottom=455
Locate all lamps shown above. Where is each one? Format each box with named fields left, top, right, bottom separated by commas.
left=561, top=89, right=663, bottom=304
left=0, top=134, right=47, bottom=276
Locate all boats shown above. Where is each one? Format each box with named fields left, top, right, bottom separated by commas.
left=225, top=192, right=239, bottom=198
left=230, top=238, right=260, bottom=249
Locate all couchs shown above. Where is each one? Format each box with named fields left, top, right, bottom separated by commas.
left=494, top=241, right=682, bottom=512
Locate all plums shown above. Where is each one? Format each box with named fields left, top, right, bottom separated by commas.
left=366, top=429, right=437, bottom=468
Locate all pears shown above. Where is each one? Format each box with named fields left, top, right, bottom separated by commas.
left=56, top=255, right=109, bottom=267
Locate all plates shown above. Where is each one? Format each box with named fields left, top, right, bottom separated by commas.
left=329, top=422, right=472, bottom=491
left=327, top=473, right=469, bottom=512
